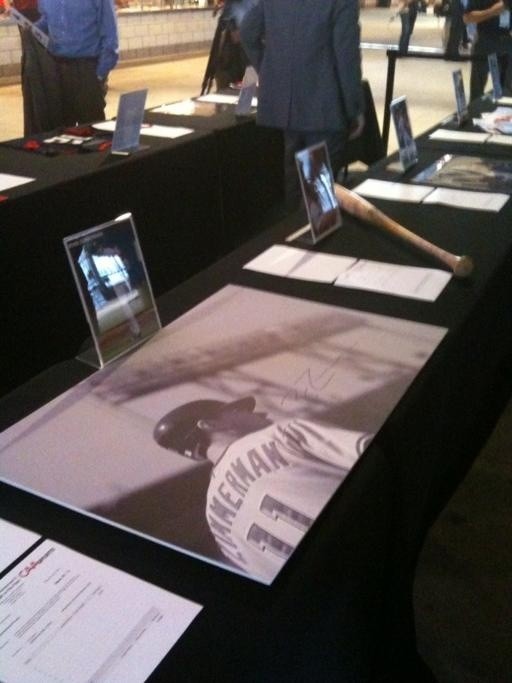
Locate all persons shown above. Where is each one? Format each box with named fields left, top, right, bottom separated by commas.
left=152, top=394, right=375, bottom=586
left=400, top=0, right=512, bottom=105
left=18, top=0, right=120, bottom=139
left=209, top=0, right=256, bottom=93
left=239, top=0, right=366, bottom=221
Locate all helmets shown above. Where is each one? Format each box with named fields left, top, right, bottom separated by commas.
left=154, top=396, right=255, bottom=462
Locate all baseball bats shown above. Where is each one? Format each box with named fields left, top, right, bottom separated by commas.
left=333, top=184, right=475, bottom=279
